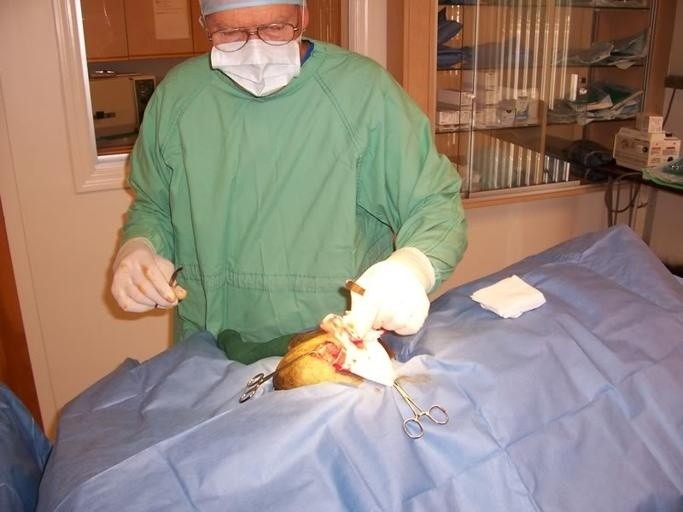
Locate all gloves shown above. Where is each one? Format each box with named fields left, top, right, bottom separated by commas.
left=350, top=246, right=435, bottom=336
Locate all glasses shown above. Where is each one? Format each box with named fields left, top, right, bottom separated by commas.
left=208, top=22, right=300, bottom=52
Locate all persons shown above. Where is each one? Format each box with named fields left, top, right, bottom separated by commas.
left=109, top=1, right=468, bottom=366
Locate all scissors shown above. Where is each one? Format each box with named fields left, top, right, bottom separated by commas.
left=238, top=352, right=310, bottom=404
left=393, top=380, right=449, bottom=440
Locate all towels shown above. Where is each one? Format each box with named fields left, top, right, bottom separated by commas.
left=470, top=274, right=547, bottom=319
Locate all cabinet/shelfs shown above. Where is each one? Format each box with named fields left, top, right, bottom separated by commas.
left=590, top=161, right=683, bottom=276
left=82, top=1, right=211, bottom=65
left=404, top=0, right=676, bottom=200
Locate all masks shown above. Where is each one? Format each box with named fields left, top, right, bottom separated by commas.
left=199, top=7, right=305, bottom=98
